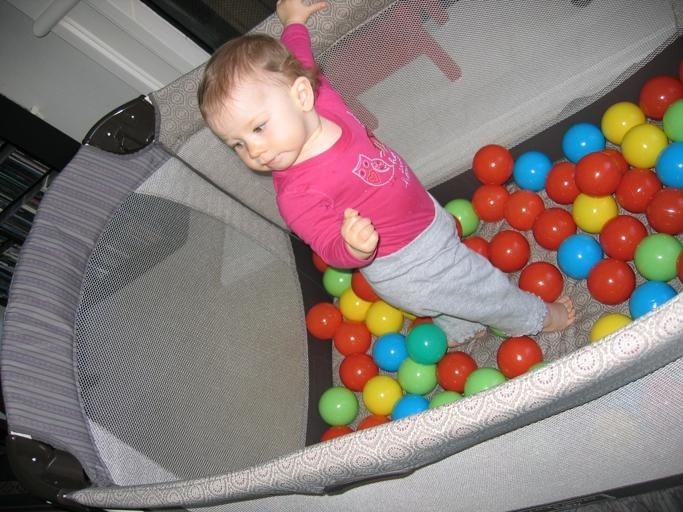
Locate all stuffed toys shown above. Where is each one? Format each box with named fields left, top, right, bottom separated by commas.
left=305, top=53, right=683, bottom=446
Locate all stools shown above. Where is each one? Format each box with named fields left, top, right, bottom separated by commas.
left=319, top=0, right=462, bottom=132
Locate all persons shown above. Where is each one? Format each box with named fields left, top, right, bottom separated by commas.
left=195, top=0, right=575, bottom=348
